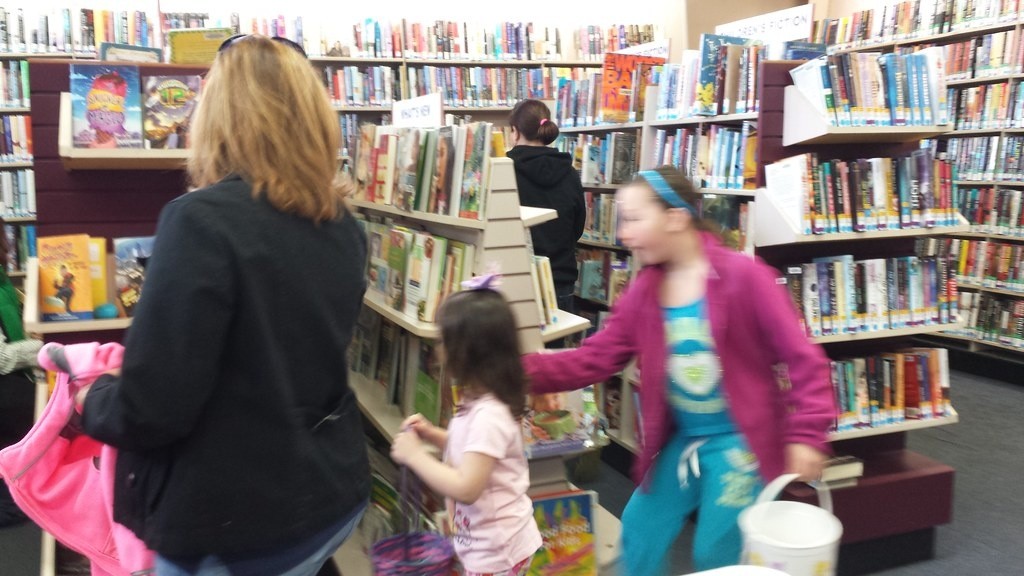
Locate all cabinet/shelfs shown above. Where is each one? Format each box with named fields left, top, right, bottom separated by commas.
left=0, top=2, right=1023, bottom=576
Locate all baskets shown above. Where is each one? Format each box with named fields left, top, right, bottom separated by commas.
left=371, top=426, right=454, bottom=576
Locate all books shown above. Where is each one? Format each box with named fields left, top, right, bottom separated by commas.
left=526, top=490, right=597, bottom=576
left=0, top=0, right=1024, bottom=556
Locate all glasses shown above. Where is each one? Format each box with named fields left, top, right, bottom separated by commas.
left=218, top=34, right=308, bottom=58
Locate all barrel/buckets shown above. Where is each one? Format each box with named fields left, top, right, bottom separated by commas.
left=369, top=428, right=456, bottom=575
left=738, top=473, right=843, bottom=576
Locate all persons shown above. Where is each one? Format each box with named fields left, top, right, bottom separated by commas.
left=390, top=288, right=543, bottom=576
left=522, top=163, right=835, bottom=576
left=53, top=264, right=75, bottom=312
left=75, top=35, right=373, bottom=576
left=504, top=100, right=587, bottom=350
